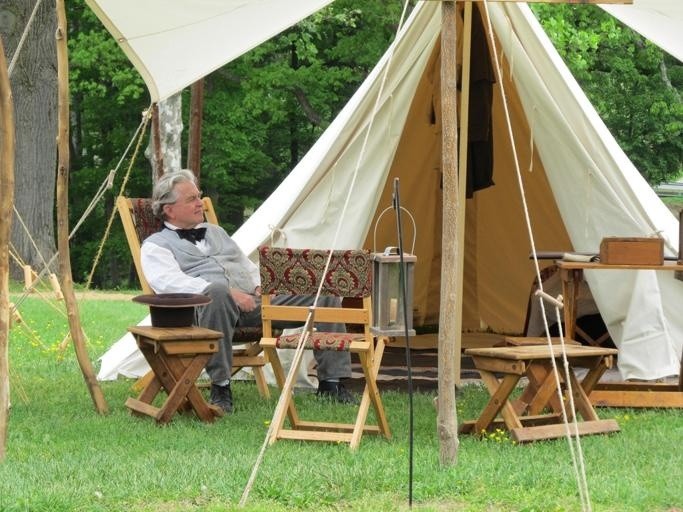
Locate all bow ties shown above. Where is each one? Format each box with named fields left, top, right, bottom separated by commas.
left=176, top=227, right=206, bottom=244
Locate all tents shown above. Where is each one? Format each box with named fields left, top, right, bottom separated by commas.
left=95, top=0, right=683, bottom=388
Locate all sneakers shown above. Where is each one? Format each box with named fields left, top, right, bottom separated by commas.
left=316, top=380, right=360, bottom=406
left=211, top=380, right=233, bottom=414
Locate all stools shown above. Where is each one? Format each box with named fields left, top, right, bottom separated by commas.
left=124, top=325, right=225, bottom=425
left=504, top=335, right=581, bottom=416
left=458, top=346, right=621, bottom=442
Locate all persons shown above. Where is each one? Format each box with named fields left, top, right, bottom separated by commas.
left=140, top=169, right=362, bottom=406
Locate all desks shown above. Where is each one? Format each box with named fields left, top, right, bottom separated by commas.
left=554, top=259, right=683, bottom=409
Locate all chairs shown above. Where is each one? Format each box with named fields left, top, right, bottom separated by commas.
left=258, top=247, right=395, bottom=452
left=114, top=195, right=283, bottom=400
